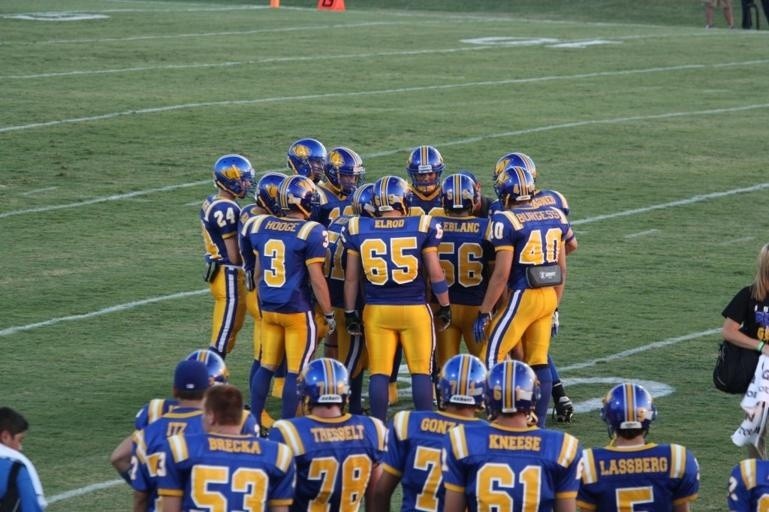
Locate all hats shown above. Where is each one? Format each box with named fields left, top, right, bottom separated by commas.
left=174, top=361, right=210, bottom=392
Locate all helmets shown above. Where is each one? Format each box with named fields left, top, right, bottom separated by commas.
left=441, top=170, right=480, bottom=213
left=600, top=383, right=658, bottom=441
left=297, top=358, right=352, bottom=403
left=407, top=145, right=444, bottom=195
left=351, top=176, right=413, bottom=218
left=436, top=354, right=488, bottom=410
left=484, top=361, right=543, bottom=426
left=492, top=152, right=536, bottom=210
left=186, top=350, right=229, bottom=389
left=213, top=154, right=256, bottom=200
left=256, top=138, right=364, bottom=219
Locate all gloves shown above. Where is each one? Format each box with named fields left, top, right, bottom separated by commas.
left=321, top=311, right=336, bottom=336
left=434, top=306, right=451, bottom=333
left=472, top=312, right=491, bottom=343
left=551, top=308, right=559, bottom=337
left=345, top=310, right=362, bottom=337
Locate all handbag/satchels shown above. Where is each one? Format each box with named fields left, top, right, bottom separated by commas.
left=713, top=324, right=760, bottom=394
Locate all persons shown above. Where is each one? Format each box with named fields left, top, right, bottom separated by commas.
left=199, top=139, right=578, bottom=438
left=111, top=352, right=698, bottom=512
left=742, top=1, right=769, bottom=30
left=706, top=0, right=734, bottom=29
left=718, top=245, right=768, bottom=512
left=1, top=406, right=47, bottom=512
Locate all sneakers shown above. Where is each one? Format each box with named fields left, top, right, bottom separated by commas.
left=556, top=397, right=574, bottom=422
